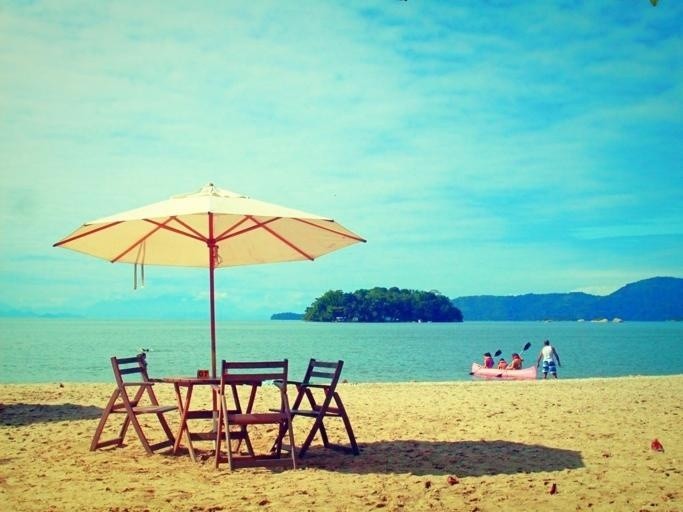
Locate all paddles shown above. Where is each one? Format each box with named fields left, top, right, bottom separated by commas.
left=470, top=350, right=501, bottom=375
left=496, top=343, right=531, bottom=377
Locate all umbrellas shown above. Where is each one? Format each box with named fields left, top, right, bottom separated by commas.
left=51, top=181, right=368, bottom=449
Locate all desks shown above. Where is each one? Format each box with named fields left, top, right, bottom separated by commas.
left=146, top=377, right=256, bottom=464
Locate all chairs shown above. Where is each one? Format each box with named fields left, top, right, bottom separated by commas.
left=269, top=358, right=360, bottom=459
left=89, top=353, right=183, bottom=457
left=210, top=358, right=297, bottom=473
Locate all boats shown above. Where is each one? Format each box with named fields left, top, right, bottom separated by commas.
left=578, top=317, right=623, bottom=323
left=472, top=363, right=537, bottom=379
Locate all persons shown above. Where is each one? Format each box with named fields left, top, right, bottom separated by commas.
left=496, top=358, right=507, bottom=370
left=479, top=352, right=494, bottom=368
left=504, top=352, right=523, bottom=369
left=536, top=339, right=560, bottom=379
